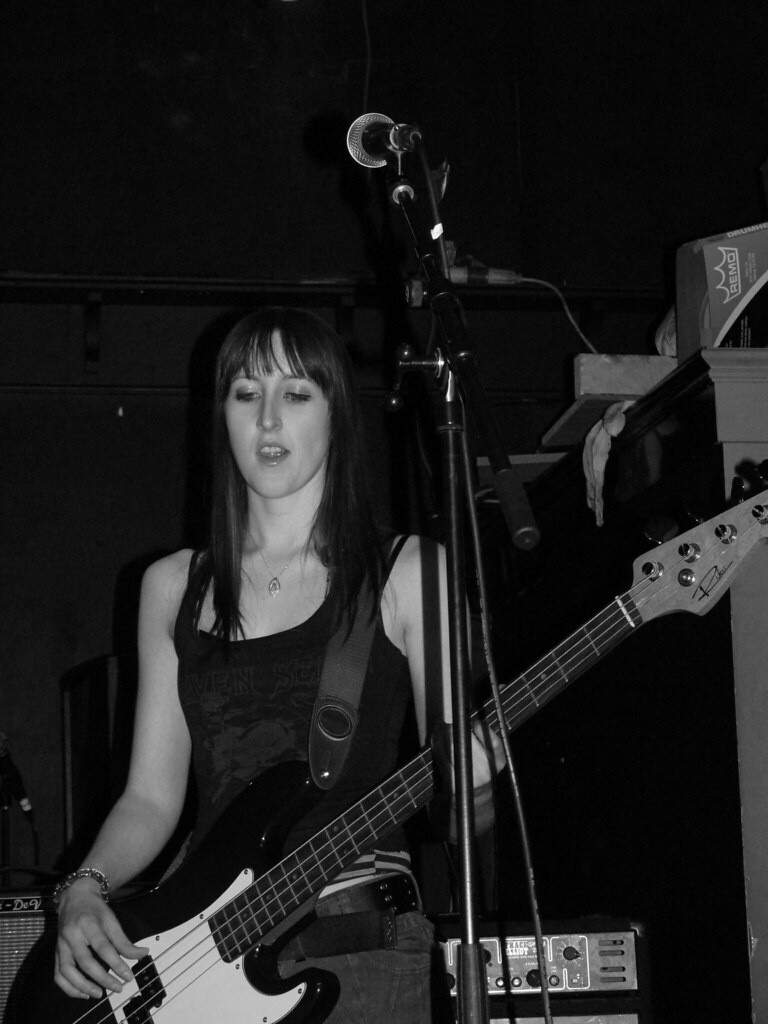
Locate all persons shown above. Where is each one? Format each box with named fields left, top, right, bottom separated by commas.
left=52, top=276, right=515, bottom=1024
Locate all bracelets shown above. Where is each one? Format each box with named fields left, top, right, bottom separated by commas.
left=50, top=869, right=112, bottom=907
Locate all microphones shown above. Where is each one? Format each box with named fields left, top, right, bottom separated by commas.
left=347, top=112, right=421, bottom=169
left=0, top=733, right=36, bottom=824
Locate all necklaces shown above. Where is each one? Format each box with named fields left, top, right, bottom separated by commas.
left=241, top=515, right=316, bottom=600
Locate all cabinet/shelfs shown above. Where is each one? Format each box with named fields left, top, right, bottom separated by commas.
left=604, top=348, right=767, bottom=1024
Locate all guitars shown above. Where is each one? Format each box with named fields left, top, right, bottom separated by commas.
left=19, top=459, right=768, bottom=1022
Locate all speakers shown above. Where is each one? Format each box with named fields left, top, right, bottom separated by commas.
left=1, top=878, right=161, bottom=1024
